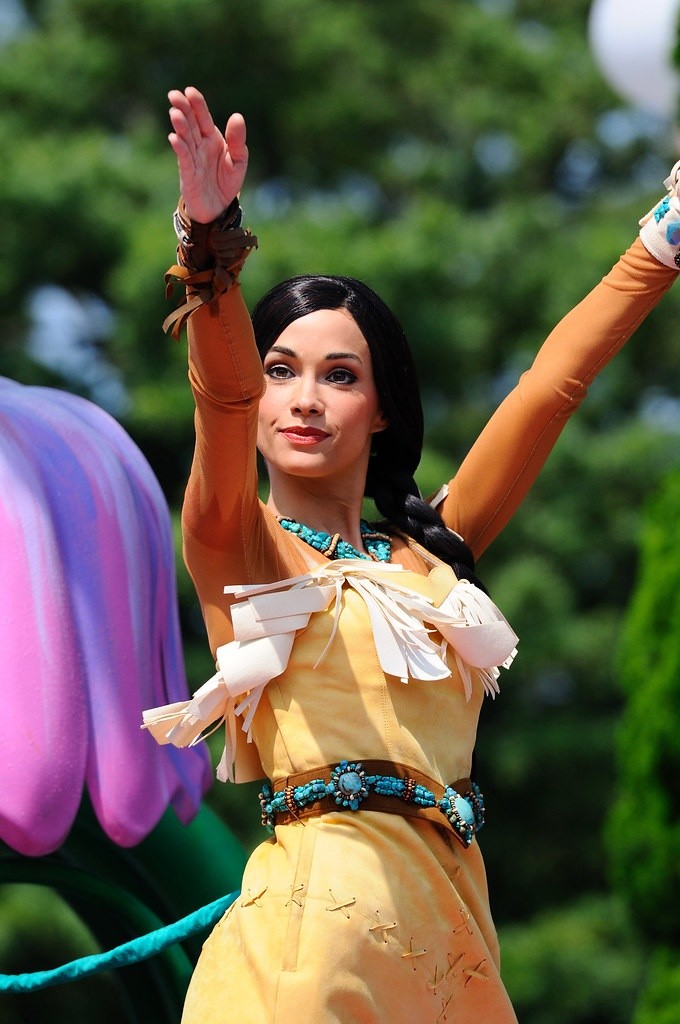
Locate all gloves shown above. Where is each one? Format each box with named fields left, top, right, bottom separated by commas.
left=639, top=159, right=680, bottom=270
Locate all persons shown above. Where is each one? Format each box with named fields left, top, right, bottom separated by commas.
left=164, top=85, right=680, bottom=1024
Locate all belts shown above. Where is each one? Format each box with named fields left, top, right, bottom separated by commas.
left=257, top=755, right=485, bottom=848
